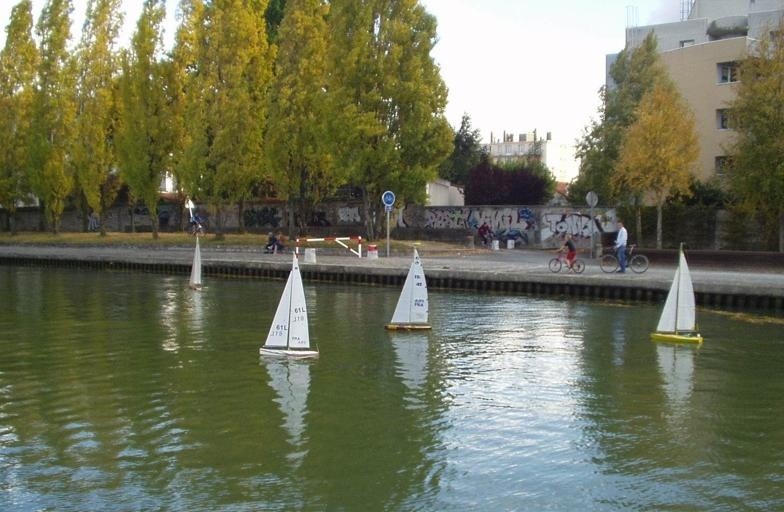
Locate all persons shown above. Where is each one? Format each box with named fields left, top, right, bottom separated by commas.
left=190, top=211, right=205, bottom=237
left=268, top=232, right=284, bottom=254
left=611, top=220, right=629, bottom=273
left=478, top=220, right=493, bottom=245
left=263, top=229, right=277, bottom=253
left=557, top=233, right=578, bottom=274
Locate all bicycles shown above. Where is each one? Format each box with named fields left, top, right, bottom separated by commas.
left=600, top=244, right=649, bottom=275
left=548, top=251, right=586, bottom=273
left=192, top=222, right=208, bottom=236
